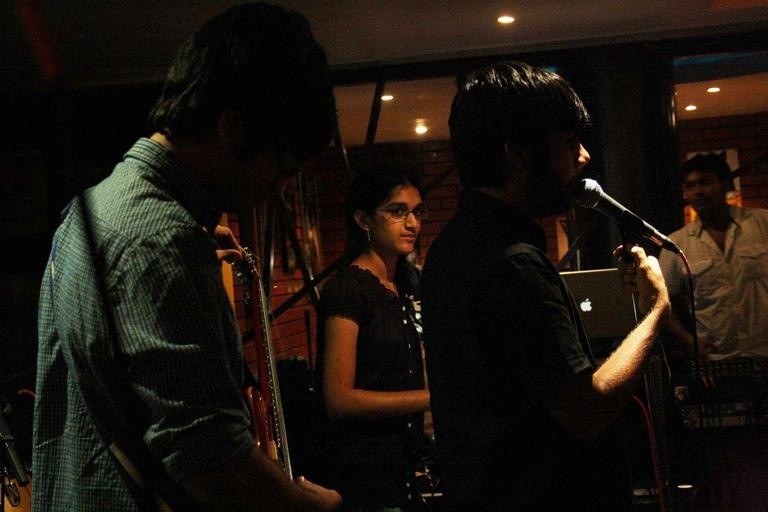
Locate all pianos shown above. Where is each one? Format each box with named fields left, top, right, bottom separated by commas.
left=675, top=387, right=767, bottom=427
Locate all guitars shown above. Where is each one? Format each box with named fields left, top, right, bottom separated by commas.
left=0, top=411, right=32, bottom=512
left=396, top=292, right=442, bottom=510
left=230, top=247, right=294, bottom=484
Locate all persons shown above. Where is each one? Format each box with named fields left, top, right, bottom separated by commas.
left=30, top=1, right=349, bottom=511
left=418, top=59, right=674, bottom=509
left=314, top=163, right=432, bottom=511
left=658, top=150, right=768, bottom=366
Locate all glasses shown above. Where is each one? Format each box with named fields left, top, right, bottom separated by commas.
left=374, top=205, right=429, bottom=222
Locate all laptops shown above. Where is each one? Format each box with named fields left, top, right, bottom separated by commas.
left=556, top=268, right=640, bottom=343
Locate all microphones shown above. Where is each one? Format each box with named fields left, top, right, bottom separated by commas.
left=574, top=178, right=680, bottom=257
